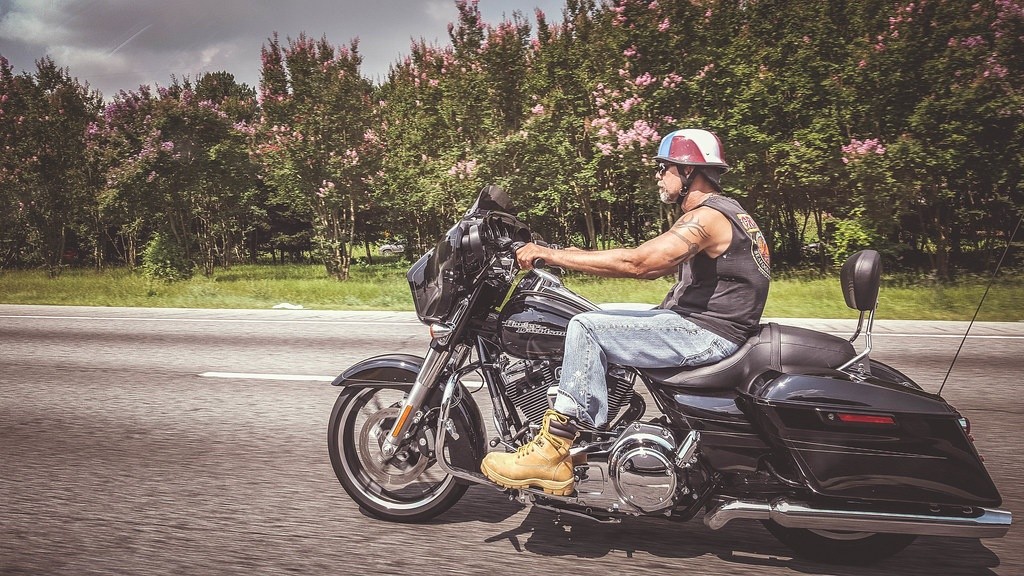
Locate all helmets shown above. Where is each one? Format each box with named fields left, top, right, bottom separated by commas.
left=652, top=129, right=729, bottom=175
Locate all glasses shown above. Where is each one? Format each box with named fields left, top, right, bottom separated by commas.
left=652, top=160, right=676, bottom=176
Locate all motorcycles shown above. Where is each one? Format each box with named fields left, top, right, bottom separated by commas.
left=326, top=184, right=1015, bottom=565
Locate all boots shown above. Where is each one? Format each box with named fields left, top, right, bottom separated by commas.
left=480, top=409, right=581, bottom=495
left=569, top=449, right=590, bottom=472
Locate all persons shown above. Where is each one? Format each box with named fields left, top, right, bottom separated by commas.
left=479, top=127, right=774, bottom=497
